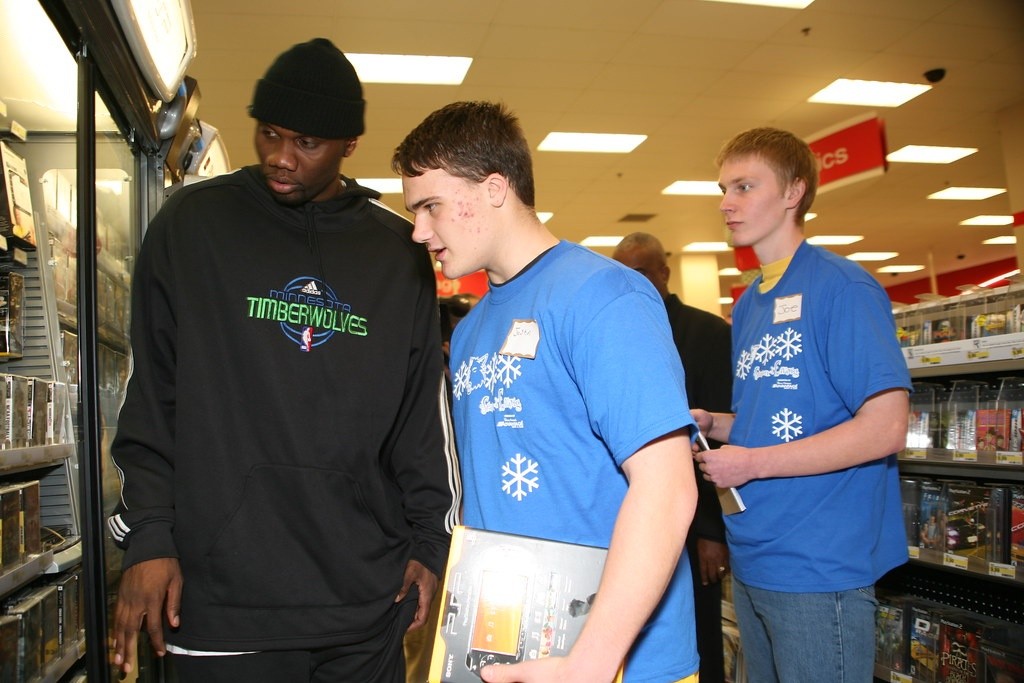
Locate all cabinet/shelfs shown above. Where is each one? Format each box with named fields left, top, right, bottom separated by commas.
left=719, top=331, right=1024, bottom=683
left=0, top=127, right=147, bottom=683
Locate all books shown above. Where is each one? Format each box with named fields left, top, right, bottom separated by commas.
left=697, top=428, right=748, bottom=515
left=426, top=525, right=624, bottom=683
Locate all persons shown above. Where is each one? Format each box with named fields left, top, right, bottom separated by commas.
left=392, top=99, right=703, bottom=683
left=689, top=128, right=913, bottom=682
left=612, top=233, right=733, bottom=682
left=108, top=36, right=463, bottom=683
left=439, top=291, right=481, bottom=401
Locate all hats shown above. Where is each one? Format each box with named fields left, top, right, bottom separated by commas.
left=248, top=38, right=366, bottom=139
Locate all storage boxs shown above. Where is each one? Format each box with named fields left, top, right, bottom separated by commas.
left=872, top=302, right=1024, bottom=683
